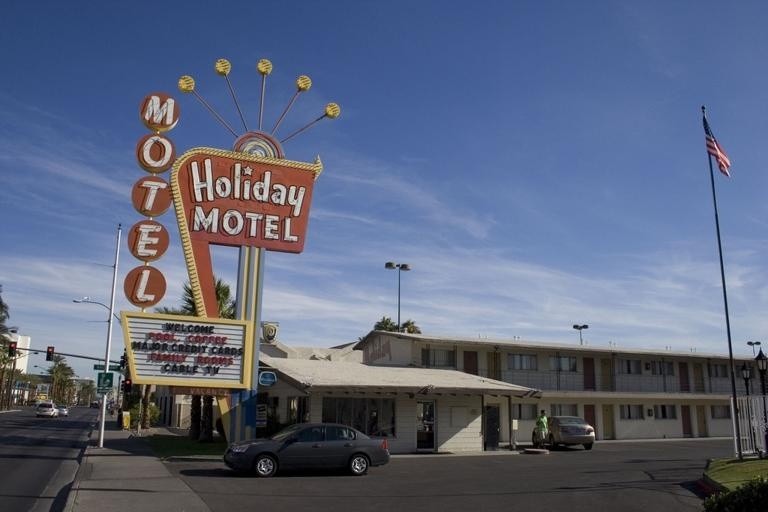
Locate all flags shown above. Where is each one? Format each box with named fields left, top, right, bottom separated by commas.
left=702, top=116, right=730, bottom=179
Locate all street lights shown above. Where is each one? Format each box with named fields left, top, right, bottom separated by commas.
left=747, top=342, right=761, bottom=356
left=740, top=361, right=756, bottom=450
left=73, top=299, right=120, bottom=321
left=754, top=348, right=768, bottom=452
left=573, top=325, right=588, bottom=345
left=385, top=262, right=412, bottom=332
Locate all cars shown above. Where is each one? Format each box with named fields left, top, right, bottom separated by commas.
left=90, top=401, right=98, bottom=408
left=224, top=423, right=390, bottom=477
left=35, top=402, right=68, bottom=418
left=532, top=416, right=595, bottom=449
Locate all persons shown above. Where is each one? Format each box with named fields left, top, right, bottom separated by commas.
left=536, top=409, right=549, bottom=448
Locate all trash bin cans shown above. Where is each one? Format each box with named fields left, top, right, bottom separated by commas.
left=117, top=409, right=131, bottom=429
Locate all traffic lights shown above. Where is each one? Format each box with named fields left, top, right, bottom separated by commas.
left=9, top=342, right=17, bottom=357
left=121, top=379, right=131, bottom=394
left=46, top=347, right=54, bottom=361
